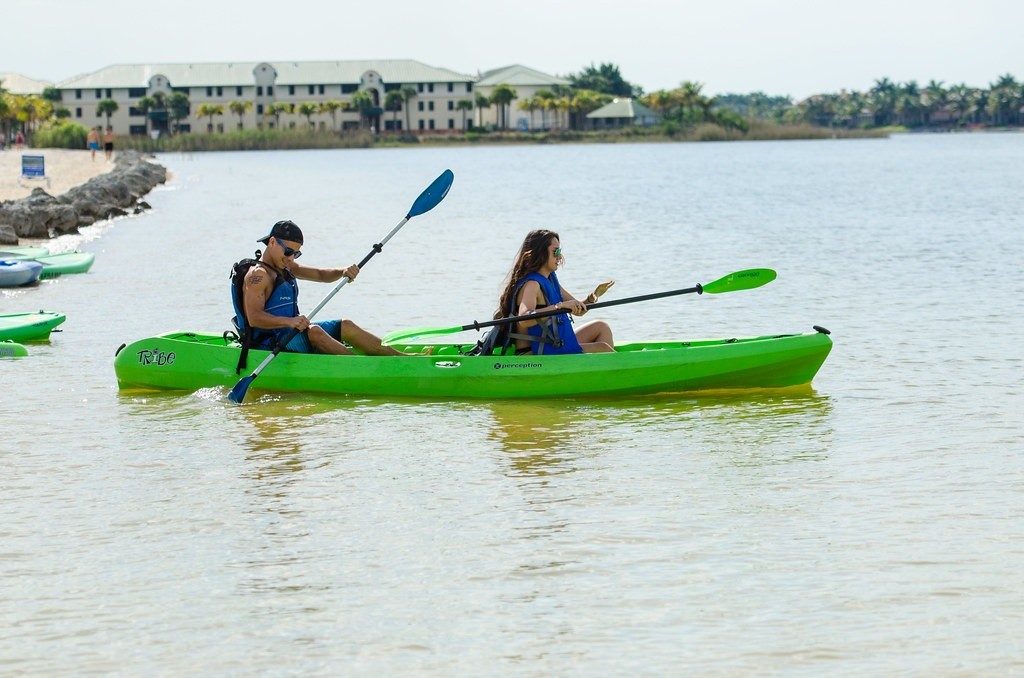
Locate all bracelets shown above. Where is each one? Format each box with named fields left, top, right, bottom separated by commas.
left=587, top=293, right=599, bottom=303
left=554, top=302, right=563, bottom=309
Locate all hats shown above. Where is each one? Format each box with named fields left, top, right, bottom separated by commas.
left=257, top=220, right=303, bottom=246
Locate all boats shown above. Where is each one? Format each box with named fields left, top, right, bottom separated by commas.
left=0, top=338, right=30, bottom=358
left=0, top=246, right=97, bottom=289
left=0, top=308, right=67, bottom=343
left=114, top=324, right=833, bottom=401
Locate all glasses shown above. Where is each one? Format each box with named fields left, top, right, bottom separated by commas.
left=275, top=238, right=302, bottom=259
left=547, top=248, right=561, bottom=257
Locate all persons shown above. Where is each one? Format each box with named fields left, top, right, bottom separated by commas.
left=15, top=132, right=24, bottom=151
left=243, top=220, right=434, bottom=355
left=493, top=229, right=617, bottom=356
left=102, top=125, right=115, bottom=161
left=87, top=128, right=100, bottom=161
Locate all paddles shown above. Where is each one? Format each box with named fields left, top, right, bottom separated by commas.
left=380, top=267, right=778, bottom=347
left=230, top=168, right=455, bottom=404
